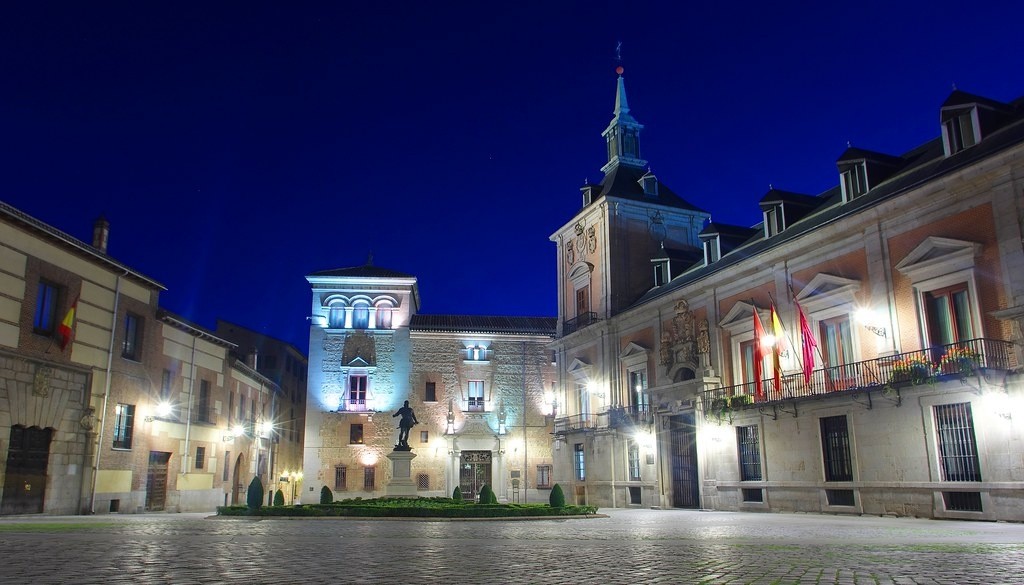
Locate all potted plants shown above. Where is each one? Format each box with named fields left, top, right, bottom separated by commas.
left=706, top=392, right=754, bottom=426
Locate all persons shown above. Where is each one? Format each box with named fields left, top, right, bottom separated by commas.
left=392, top=400, right=419, bottom=448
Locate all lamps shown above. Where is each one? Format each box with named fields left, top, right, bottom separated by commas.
left=223, top=425, right=245, bottom=442
left=145, top=402, right=172, bottom=422
left=587, top=382, right=605, bottom=399
left=763, top=335, right=790, bottom=359
left=544, top=392, right=562, bottom=407
left=635, top=432, right=652, bottom=448
left=257, top=422, right=273, bottom=438
left=856, top=307, right=886, bottom=338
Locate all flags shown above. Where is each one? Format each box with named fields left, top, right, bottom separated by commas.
left=794, top=295, right=817, bottom=384
left=754, top=306, right=773, bottom=402
left=770, top=299, right=786, bottom=393
left=56, top=295, right=79, bottom=352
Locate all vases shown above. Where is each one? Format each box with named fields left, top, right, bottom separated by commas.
left=893, top=367, right=929, bottom=382
left=940, top=362, right=978, bottom=374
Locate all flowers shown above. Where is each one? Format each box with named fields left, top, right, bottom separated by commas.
left=934, top=344, right=983, bottom=376
left=882, top=352, right=939, bottom=394
